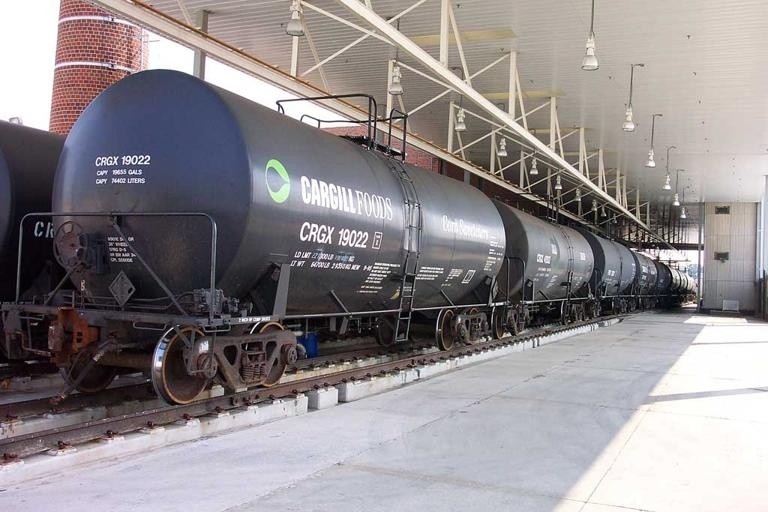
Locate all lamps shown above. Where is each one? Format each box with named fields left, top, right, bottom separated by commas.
left=619, top=54, right=647, bottom=137
left=389, top=13, right=403, bottom=96
left=454, top=65, right=466, bottom=132
left=285, top=1, right=305, bottom=37
left=496, top=102, right=509, bottom=156
left=580, top=0, right=600, bottom=72
left=645, top=114, right=693, bottom=221
left=528, top=126, right=618, bottom=224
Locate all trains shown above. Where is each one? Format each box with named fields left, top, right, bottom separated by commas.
left=0, top=57, right=702, bottom=417
left=0, top=112, right=65, bottom=381
